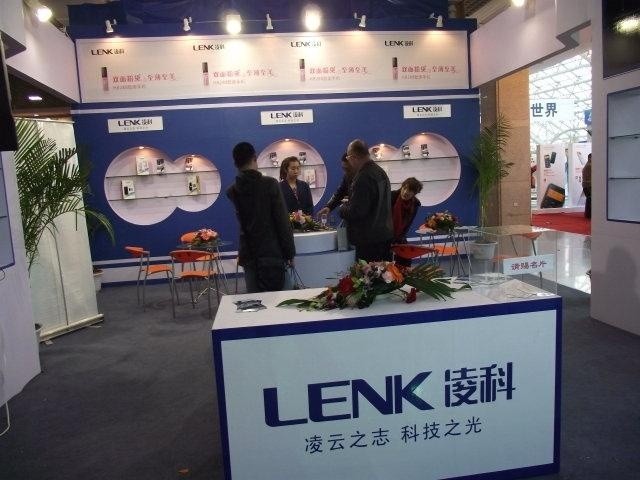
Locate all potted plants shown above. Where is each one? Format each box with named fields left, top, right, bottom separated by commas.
left=462, top=109, right=513, bottom=262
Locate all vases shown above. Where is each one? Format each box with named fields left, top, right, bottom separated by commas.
left=93, top=269, right=104, bottom=295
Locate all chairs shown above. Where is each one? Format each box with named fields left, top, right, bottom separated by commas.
left=416, top=221, right=479, bottom=277
left=125, top=229, right=229, bottom=319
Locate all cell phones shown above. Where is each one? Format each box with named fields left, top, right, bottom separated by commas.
left=544, top=151, right=557, bottom=168
left=101, top=57, right=399, bottom=93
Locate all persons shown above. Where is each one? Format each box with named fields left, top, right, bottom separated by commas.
left=391, top=176, right=424, bottom=267
left=224, top=141, right=297, bottom=292
left=278, top=155, right=313, bottom=214
left=338, top=137, right=395, bottom=263
left=317, top=153, right=357, bottom=227
left=582, top=152, right=591, bottom=219
